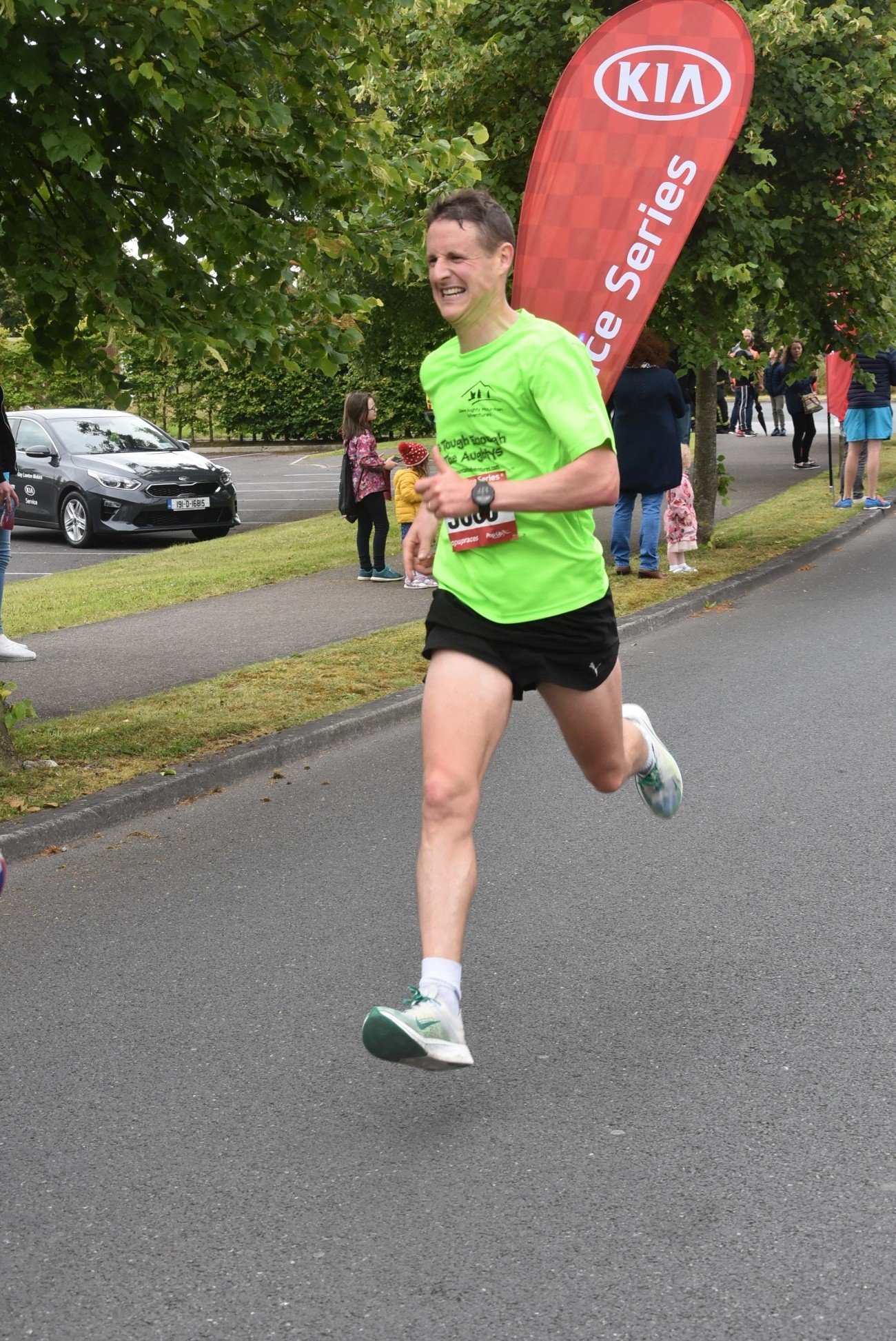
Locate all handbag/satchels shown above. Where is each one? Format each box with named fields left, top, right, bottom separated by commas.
left=800, top=391, right=824, bottom=414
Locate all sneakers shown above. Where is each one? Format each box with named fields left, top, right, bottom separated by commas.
left=357, top=567, right=374, bottom=581
left=863, top=495, right=891, bottom=510
left=833, top=498, right=853, bottom=509
left=622, top=703, right=682, bottom=820
left=370, top=566, right=404, bottom=581
left=362, top=985, right=474, bottom=1072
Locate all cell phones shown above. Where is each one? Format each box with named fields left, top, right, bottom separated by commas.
left=392, top=456, right=402, bottom=463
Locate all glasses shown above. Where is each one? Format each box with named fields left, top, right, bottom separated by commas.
left=368, top=406, right=375, bottom=412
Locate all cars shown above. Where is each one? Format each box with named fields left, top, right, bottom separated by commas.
left=4, top=408, right=246, bottom=546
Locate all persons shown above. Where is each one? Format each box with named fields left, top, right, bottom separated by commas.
left=358, top=193, right=682, bottom=1071
left=664, top=443, right=699, bottom=575
left=669, top=329, right=896, bottom=510
left=0, top=386, right=37, bottom=661
left=605, top=327, right=683, bottom=579
left=394, top=442, right=439, bottom=590
left=339, top=394, right=405, bottom=582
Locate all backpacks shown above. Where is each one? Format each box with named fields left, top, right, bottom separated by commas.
left=338, top=438, right=364, bottom=523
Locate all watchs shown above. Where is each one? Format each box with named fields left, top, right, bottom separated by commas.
left=471, top=480, right=494, bottom=519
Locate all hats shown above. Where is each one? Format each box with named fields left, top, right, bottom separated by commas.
left=398, top=441, right=429, bottom=467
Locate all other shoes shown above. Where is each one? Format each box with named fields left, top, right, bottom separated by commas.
left=404, top=576, right=427, bottom=589
left=771, top=428, right=787, bottom=436
left=0, top=634, right=36, bottom=661
left=735, top=429, right=759, bottom=437
left=638, top=569, right=669, bottom=580
left=728, top=427, right=736, bottom=434
left=793, top=458, right=820, bottom=470
left=416, top=574, right=438, bottom=587
left=670, top=563, right=698, bottom=574
left=615, top=566, right=630, bottom=575
left=853, top=496, right=869, bottom=503
